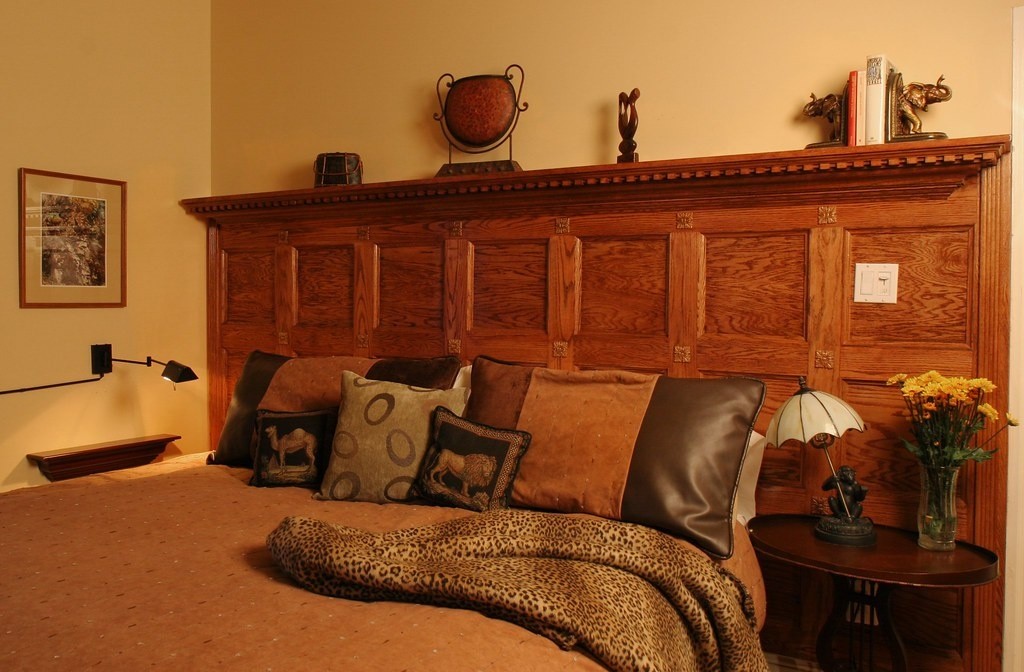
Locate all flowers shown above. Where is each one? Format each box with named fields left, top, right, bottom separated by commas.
left=887, top=370, right=1020, bottom=471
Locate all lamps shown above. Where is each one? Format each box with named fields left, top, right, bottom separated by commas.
left=765, top=376, right=879, bottom=546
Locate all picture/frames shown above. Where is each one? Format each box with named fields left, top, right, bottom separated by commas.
left=18, top=167, right=126, bottom=307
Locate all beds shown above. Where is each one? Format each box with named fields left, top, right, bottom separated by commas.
left=0, top=137, right=1013, bottom=672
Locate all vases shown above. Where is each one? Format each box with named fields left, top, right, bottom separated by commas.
left=916, top=460, right=961, bottom=551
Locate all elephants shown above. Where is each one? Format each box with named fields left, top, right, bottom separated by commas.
left=900, top=75, right=952, bottom=134
left=802, top=92, right=842, bottom=142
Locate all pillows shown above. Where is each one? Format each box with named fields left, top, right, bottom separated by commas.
left=206, top=348, right=461, bottom=468
left=312, top=368, right=472, bottom=504
left=404, top=406, right=533, bottom=513
left=464, top=355, right=767, bottom=560
left=248, top=405, right=341, bottom=488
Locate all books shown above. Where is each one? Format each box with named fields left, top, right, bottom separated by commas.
left=847, top=57, right=895, bottom=147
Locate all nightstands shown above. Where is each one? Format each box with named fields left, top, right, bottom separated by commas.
left=747, top=514, right=1000, bottom=672
